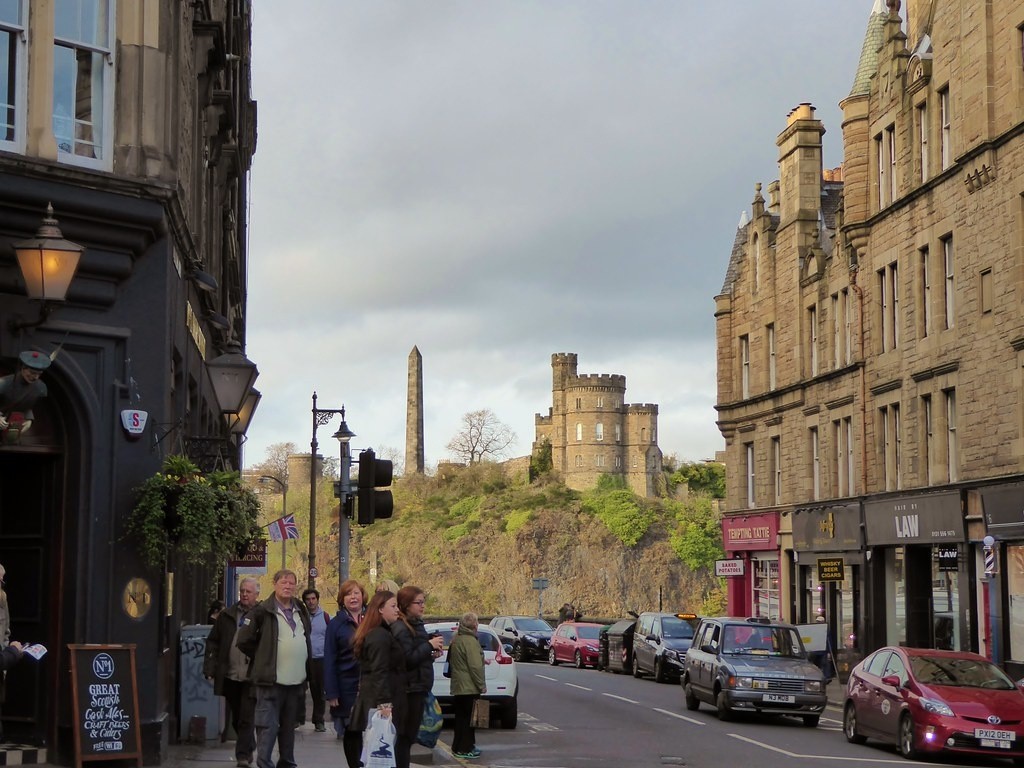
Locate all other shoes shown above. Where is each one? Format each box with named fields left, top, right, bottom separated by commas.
left=315, top=723, right=326, bottom=733
left=451, top=745, right=481, bottom=758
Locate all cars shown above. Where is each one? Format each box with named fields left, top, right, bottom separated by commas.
left=842, top=645, right=1024, bottom=768
left=490, top=615, right=556, bottom=663
left=548, top=623, right=605, bottom=669
left=425, top=622, right=519, bottom=730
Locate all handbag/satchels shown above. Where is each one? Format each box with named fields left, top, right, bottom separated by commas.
left=361, top=707, right=396, bottom=768
left=415, top=691, right=442, bottom=749
left=470, top=698, right=489, bottom=730
left=443, top=643, right=453, bottom=678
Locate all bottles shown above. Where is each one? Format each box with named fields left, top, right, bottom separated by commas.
left=431, top=629, right=443, bottom=657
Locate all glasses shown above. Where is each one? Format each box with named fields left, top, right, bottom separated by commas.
left=410, top=600, right=425, bottom=606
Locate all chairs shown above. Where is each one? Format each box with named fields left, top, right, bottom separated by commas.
left=747, top=634, right=763, bottom=648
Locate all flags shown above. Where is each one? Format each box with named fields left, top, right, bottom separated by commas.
left=268, top=514, right=300, bottom=542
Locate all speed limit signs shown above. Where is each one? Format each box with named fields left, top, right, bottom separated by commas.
left=309, top=567, right=318, bottom=577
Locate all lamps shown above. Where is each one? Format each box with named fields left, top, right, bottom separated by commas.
left=182, top=331, right=261, bottom=474
left=185, top=260, right=219, bottom=293
left=8, top=201, right=87, bottom=337
left=199, top=308, right=233, bottom=331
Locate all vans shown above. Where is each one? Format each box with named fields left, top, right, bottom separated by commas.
left=632, top=612, right=708, bottom=684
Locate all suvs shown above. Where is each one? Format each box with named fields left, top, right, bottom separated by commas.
left=680, top=616, right=828, bottom=728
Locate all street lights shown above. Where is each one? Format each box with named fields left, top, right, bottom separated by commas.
left=307, top=390, right=357, bottom=589
left=257, top=475, right=288, bottom=569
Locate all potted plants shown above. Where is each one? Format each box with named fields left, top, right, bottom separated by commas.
left=108, top=453, right=264, bottom=612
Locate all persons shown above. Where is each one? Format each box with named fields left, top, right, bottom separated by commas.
left=202, top=577, right=261, bottom=768
left=557, top=603, right=583, bottom=622
left=322, top=579, right=369, bottom=720
left=342, top=591, right=406, bottom=768
left=445, top=612, right=487, bottom=759
left=0, top=563, right=26, bottom=742
left=390, top=585, right=444, bottom=768
left=236, top=569, right=312, bottom=768
left=0, top=350, right=52, bottom=435
left=297, top=589, right=334, bottom=731
left=374, top=580, right=401, bottom=599
left=206, top=600, right=226, bottom=625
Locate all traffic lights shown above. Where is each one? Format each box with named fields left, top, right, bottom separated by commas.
left=358, top=451, right=393, bottom=523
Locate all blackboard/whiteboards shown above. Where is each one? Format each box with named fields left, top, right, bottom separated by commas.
left=67, top=644, right=143, bottom=761
